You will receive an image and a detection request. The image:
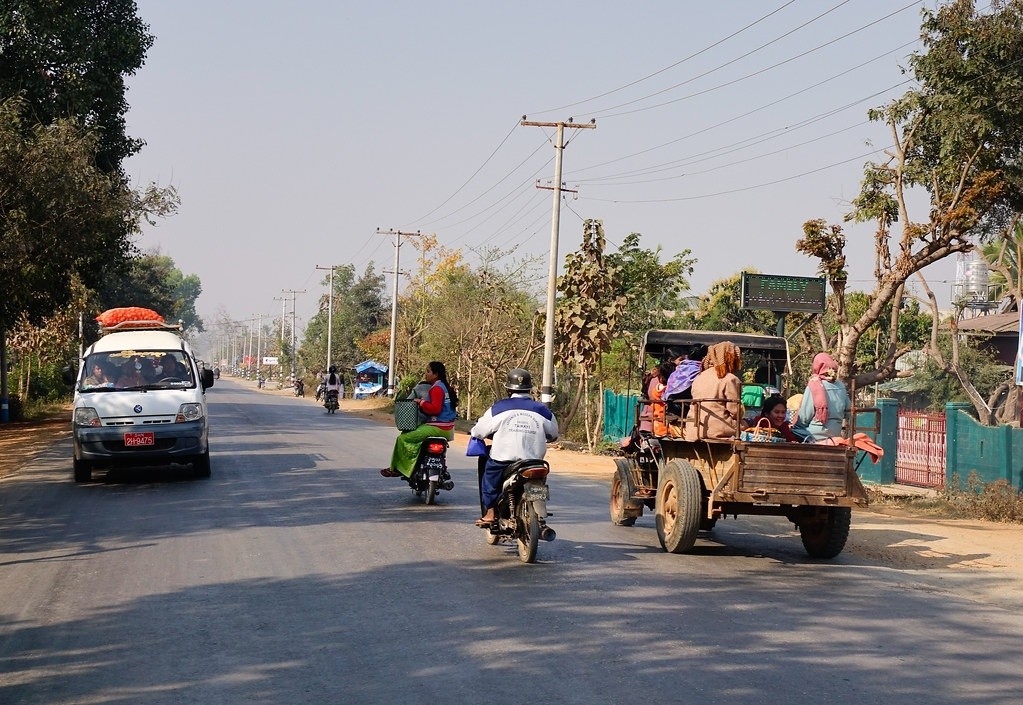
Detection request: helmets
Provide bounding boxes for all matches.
[330,366,337,373]
[505,369,533,391]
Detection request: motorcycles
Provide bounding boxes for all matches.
[258,377,265,389]
[401,399,462,506]
[467,430,556,563]
[292,375,305,397]
[315,380,325,402]
[215,367,221,380]
[319,378,342,413]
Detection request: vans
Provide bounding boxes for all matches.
[72,330,214,482]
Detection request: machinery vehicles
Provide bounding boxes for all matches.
[609,328,882,558]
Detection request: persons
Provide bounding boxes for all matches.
[208,366,370,410]
[83,354,188,389]
[644,340,850,443]
[470,369,559,525]
[406,377,432,425]
[380,362,461,477]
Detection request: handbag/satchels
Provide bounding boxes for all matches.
[739,417,786,443]
[466,437,487,456]
[393,389,420,430]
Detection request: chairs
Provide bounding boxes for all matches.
[161,363,187,381]
[102,362,117,376]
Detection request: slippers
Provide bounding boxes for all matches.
[475,517,494,524]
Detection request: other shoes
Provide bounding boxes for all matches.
[381,468,401,477]
